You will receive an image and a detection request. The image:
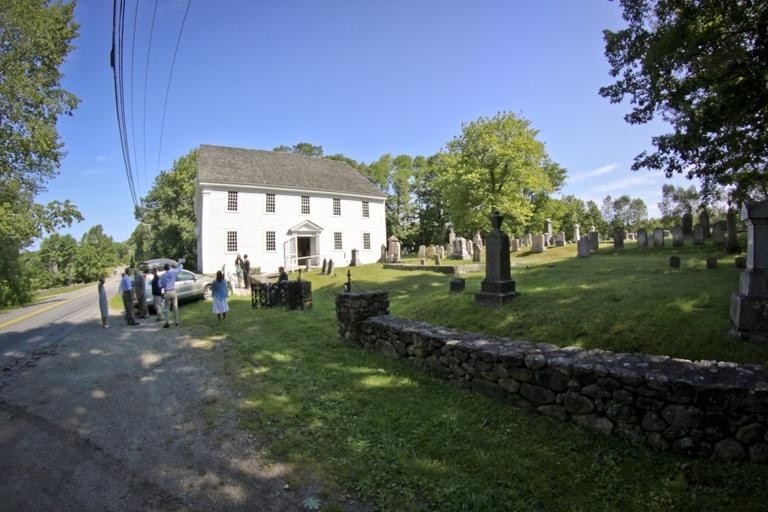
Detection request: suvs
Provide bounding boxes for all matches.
[131,258,216,309]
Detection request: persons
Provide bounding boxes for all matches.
[118,273,128,321]
[235,254,244,288]
[121,268,141,325]
[210,271,230,320]
[134,268,150,320]
[242,255,250,289]
[158,262,184,328]
[151,268,166,321]
[98,275,112,328]
[276,267,288,293]
